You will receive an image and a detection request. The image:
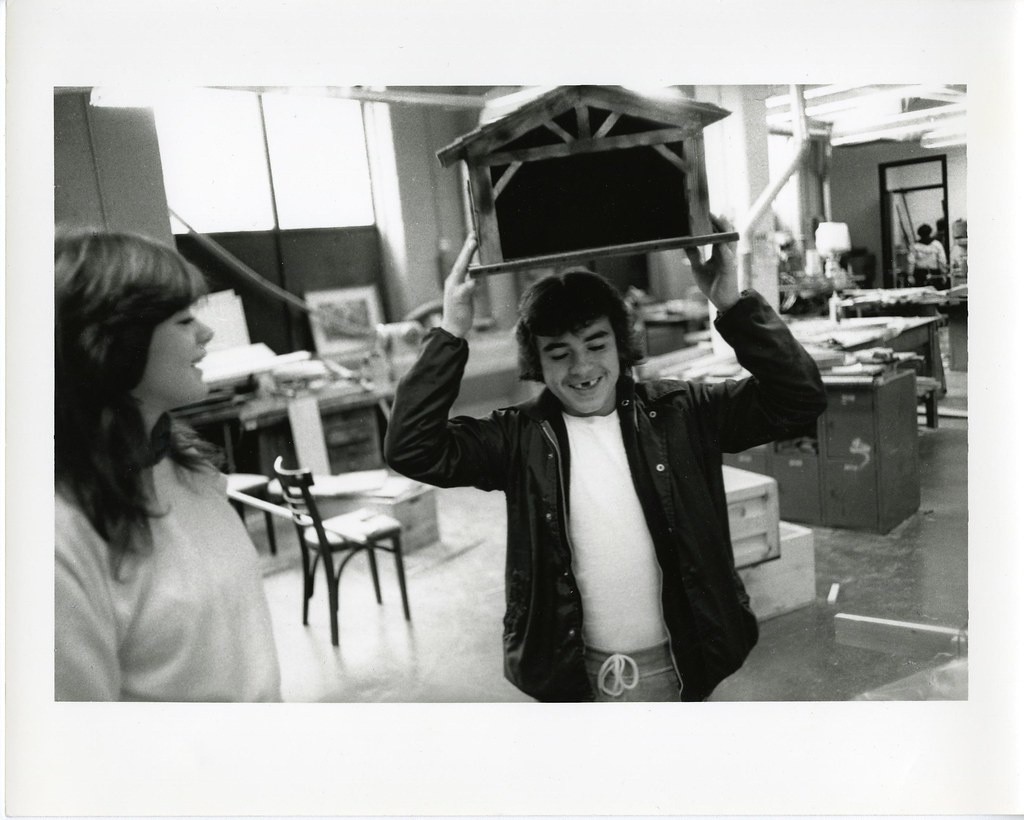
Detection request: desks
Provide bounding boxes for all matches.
[177,383,398,480]
[223,474,278,557]
[643,285,940,428]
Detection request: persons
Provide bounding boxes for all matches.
[54,230,279,703]
[908,225,947,290]
[385,209,826,702]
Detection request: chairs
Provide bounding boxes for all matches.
[274,456,412,646]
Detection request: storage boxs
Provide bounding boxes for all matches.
[363,478,439,555]
[722,464,816,622]
[321,408,382,475]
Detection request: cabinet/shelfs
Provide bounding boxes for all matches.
[722,369,922,534]
